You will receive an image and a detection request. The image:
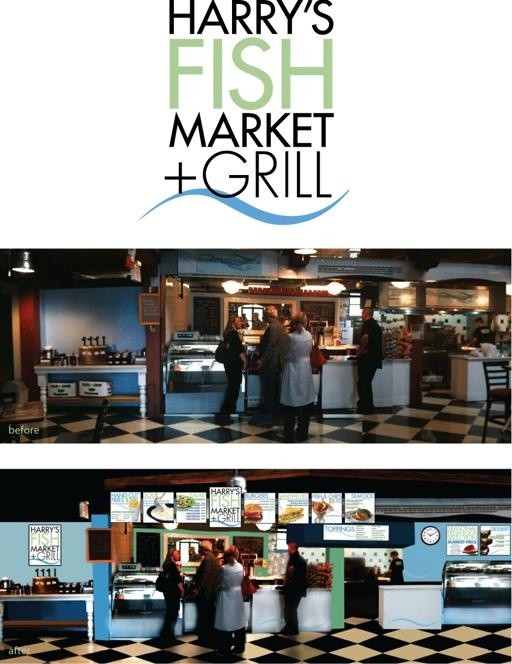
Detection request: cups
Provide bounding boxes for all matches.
[108,351,130,364]
[59,583,78,593]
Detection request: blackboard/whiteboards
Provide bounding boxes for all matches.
[190,293,224,337]
[86,528,112,562]
[139,292,161,325]
[233,535,264,557]
[297,297,339,326]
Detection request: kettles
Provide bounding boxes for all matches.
[40,350,54,359]
[0,577,9,589]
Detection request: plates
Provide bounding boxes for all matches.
[176,495,195,511]
[349,511,372,524]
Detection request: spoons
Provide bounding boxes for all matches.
[154,493,166,504]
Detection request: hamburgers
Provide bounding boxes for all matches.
[244,503,264,524]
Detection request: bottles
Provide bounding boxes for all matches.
[24,583,30,595]
[70,352,77,366]
[15,583,21,594]
[62,353,67,365]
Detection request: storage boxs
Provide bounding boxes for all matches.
[46,381,76,399]
[77,379,113,399]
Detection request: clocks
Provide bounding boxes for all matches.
[420,526,441,545]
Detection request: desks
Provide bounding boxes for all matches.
[377,584,443,632]
[1,593,94,645]
[449,353,511,402]
[33,362,147,420]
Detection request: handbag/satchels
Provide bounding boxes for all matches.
[215,342,230,363]
[310,345,326,368]
[242,576,256,595]
[155,572,166,592]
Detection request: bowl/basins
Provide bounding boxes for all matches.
[147,503,174,522]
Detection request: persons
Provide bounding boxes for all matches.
[176,538,247,654]
[155,548,187,646]
[280,543,308,636]
[470,317,495,347]
[211,305,319,443]
[381,550,405,584]
[355,305,384,415]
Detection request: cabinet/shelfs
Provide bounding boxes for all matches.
[111,565,333,641]
[441,558,512,625]
[165,338,411,424]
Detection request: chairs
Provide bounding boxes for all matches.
[481,356,511,441]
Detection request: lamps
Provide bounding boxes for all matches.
[220,277,247,295]
[391,280,411,290]
[12,249,35,274]
[323,280,347,296]
[300,284,346,291]
[249,284,270,288]
[162,523,178,530]
[294,249,319,254]
[229,470,248,494]
[255,524,273,531]
